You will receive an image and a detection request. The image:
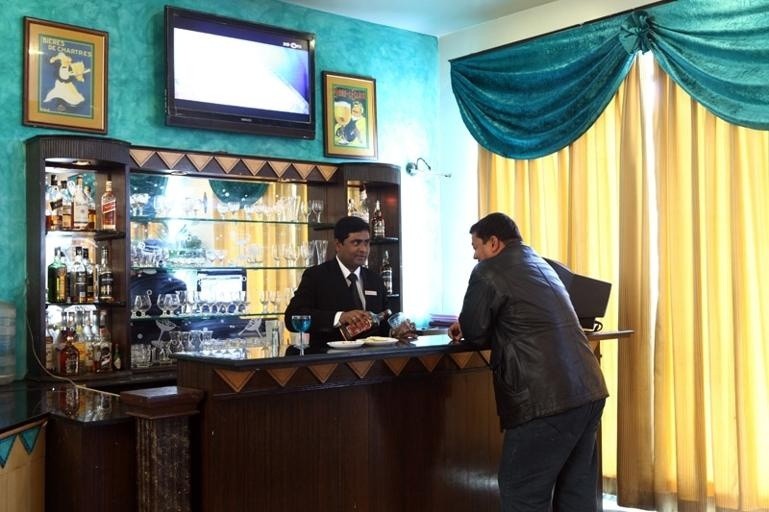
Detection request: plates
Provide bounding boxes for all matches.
[357,338,399,346]
[431,314,459,323]
[327,342,364,348]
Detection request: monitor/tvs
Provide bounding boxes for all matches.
[542,256,612,331]
[162,2,316,143]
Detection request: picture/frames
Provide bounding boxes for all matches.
[21,14,109,136]
[320,70,380,162]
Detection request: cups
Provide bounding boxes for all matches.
[131,344,153,367]
[387,312,412,337]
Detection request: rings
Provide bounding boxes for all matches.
[351,316,357,320]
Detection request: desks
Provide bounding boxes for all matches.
[0,386,47,511]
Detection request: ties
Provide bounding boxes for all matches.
[347,273,363,310]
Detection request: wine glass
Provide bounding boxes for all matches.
[202,338,248,358]
[290,315,312,349]
[130,193,329,319]
[151,319,175,348]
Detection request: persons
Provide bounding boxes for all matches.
[284,216,416,348]
[447,214,610,512]
[129,239,191,343]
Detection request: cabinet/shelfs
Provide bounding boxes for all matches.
[21,135,403,385]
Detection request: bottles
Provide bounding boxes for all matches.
[159,349,165,361]
[347,183,393,295]
[167,331,214,351]
[45,172,122,376]
[47,384,112,421]
[339,309,392,341]
